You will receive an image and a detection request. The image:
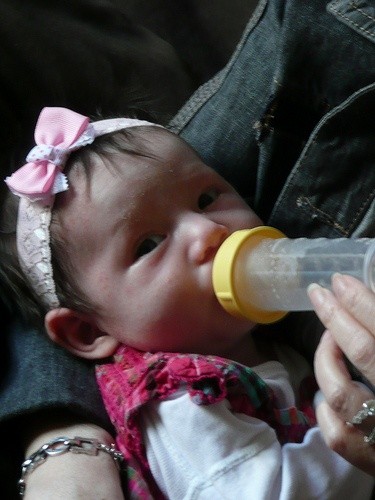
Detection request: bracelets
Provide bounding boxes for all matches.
[18,437,125,495]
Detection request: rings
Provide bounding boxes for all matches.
[345,400,375,444]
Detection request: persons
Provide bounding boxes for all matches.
[0,0,375,500]
[1,112,375,500]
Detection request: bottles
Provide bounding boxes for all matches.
[212,225,374,324]
